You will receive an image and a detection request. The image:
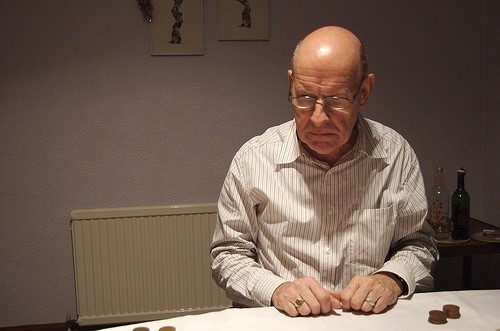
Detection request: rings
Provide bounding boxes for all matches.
[294,297,303,308]
[365,298,375,307]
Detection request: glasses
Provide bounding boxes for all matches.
[288,73,365,110]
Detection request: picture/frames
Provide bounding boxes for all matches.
[149,0,206,56]
[217,0,271,41]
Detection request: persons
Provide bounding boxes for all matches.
[210,25,439,318]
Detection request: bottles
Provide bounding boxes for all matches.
[451,169,470,241]
[429,166,450,241]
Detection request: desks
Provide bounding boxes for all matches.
[428,213,500,289]
[97,281,500,331]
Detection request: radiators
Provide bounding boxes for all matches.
[68,201,242,327]
